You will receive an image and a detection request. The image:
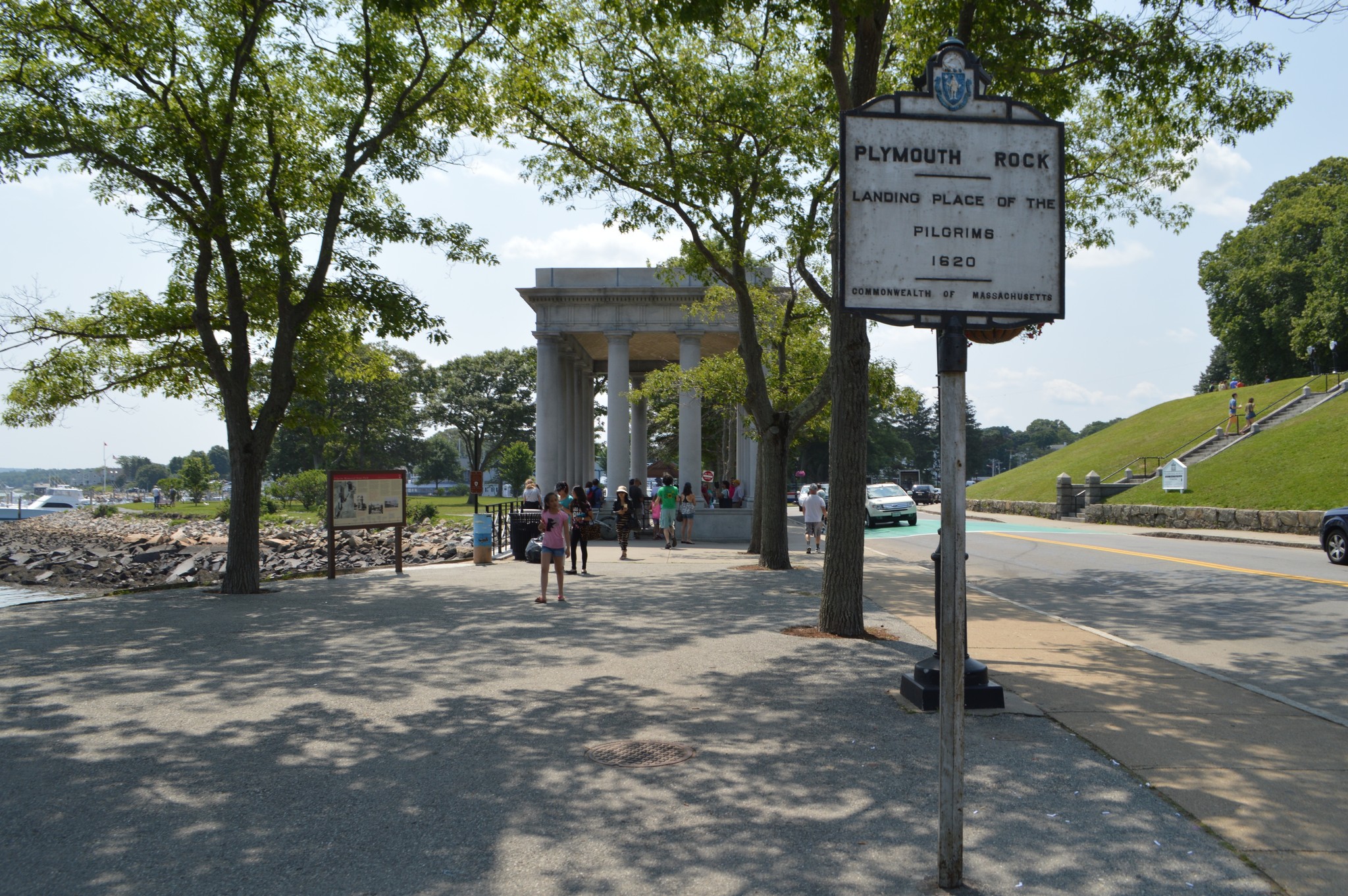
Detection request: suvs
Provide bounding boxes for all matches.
[865,482,917,529]
[910,484,939,505]
[820,483,829,507]
[796,484,811,512]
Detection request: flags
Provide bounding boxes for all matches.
[113,456,118,460]
[104,443,107,446]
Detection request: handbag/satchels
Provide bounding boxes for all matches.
[522,503,526,509]
[719,489,732,508]
[579,515,602,541]
[676,511,683,522]
[641,502,645,515]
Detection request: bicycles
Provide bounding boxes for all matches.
[569,500,619,541]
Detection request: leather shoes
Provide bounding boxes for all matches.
[581,571,587,574]
[565,571,577,574]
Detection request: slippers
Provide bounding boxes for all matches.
[558,596,565,601]
[534,597,547,603]
[620,555,626,559]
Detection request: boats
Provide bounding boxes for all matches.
[0,441,297,519]
[405,436,536,496]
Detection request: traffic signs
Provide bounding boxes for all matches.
[470,471,483,495]
[839,108,1065,321]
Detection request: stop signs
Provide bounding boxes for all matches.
[702,470,714,483]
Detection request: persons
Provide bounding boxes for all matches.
[87,484,176,508]
[653,474,680,549]
[1224,393,1245,436]
[680,482,696,544]
[647,493,663,540]
[801,483,828,554]
[534,492,571,603]
[1264,375,1270,383]
[554,481,574,572]
[565,485,594,574]
[629,477,645,539]
[585,477,744,509]
[523,479,541,510]
[1239,397,1256,435]
[611,486,635,560]
[816,483,826,534]
[535,484,544,510]
[1208,378,1243,393]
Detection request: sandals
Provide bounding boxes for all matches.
[665,543,671,549]
[671,536,677,547]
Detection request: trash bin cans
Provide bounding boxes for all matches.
[472,514,493,563]
[508,513,542,561]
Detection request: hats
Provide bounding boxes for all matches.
[634,478,642,485]
[730,477,735,479]
[1211,377,1236,384]
[554,481,565,492]
[732,479,740,484]
[684,482,692,488]
[809,483,818,491]
[616,486,629,496]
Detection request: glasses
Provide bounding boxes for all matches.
[556,488,562,492]
[618,492,625,494]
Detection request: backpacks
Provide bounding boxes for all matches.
[587,487,599,505]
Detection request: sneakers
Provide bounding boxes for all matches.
[806,546,811,554]
[815,548,821,553]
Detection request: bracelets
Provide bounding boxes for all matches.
[538,529,543,531]
[561,506,564,509]
[582,517,584,520]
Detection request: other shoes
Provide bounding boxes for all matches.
[1240,431,1245,435]
[681,540,686,543]
[686,541,695,544]
[638,520,641,526]
[1224,433,1231,436]
[633,533,664,540]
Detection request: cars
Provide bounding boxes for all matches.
[1319,508,1348,565]
[934,488,941,503]
[965,481,976,488]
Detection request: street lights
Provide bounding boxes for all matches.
[986,458,999,477]
[975,469,979,483]
[934,465,941,488]
[793,453,799,505]
[996,466,1006,474]
